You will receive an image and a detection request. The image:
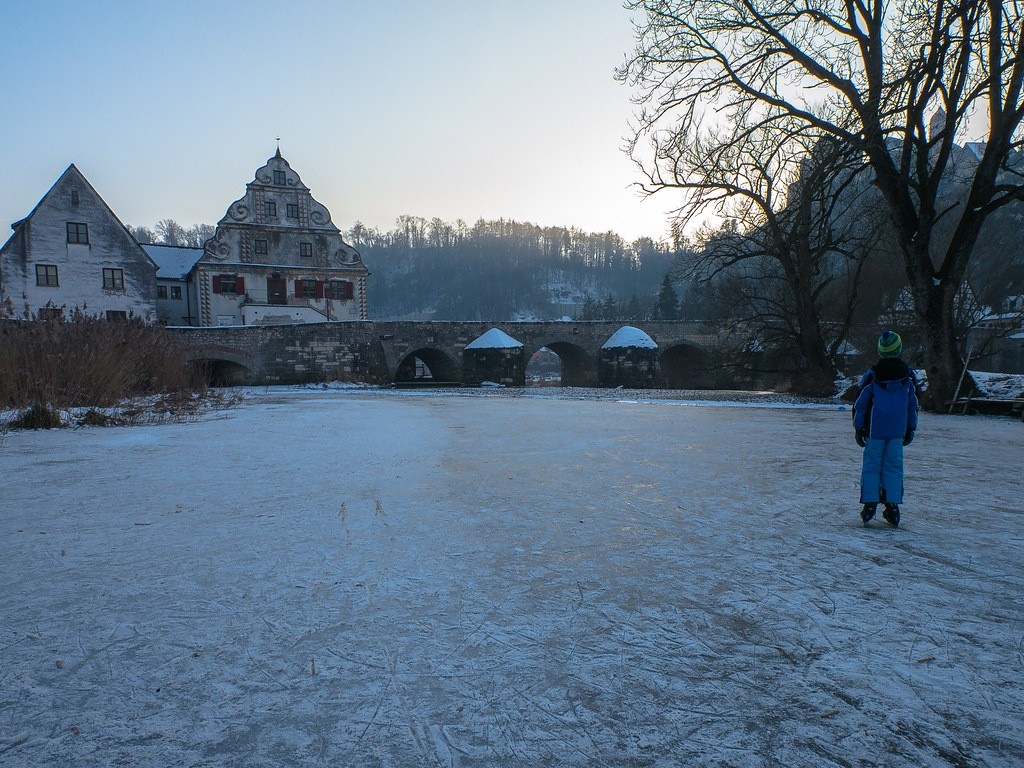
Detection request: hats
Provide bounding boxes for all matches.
[877,330,902,358]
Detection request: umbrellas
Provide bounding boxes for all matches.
[463,328,524,350]
[601,325,659,350]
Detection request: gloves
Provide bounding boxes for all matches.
[855,429,868,447]
[903,431,914,446]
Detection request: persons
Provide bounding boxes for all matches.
[852,331,921,528]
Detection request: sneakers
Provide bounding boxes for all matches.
[881,504,900,528]
[860,503,877,526]
